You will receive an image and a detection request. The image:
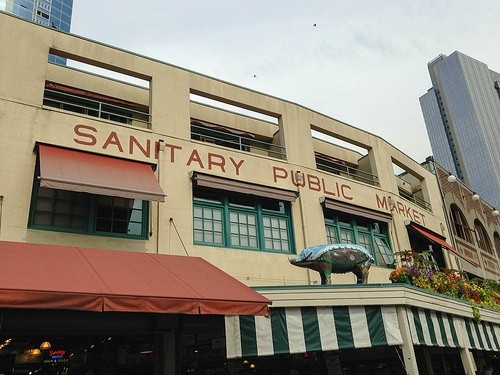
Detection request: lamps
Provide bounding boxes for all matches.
[438,172,455,184]
[388,195,394,217]
[484,207,499,217]
[295,171,301,197]
[31,349,41,356]
[468,191,479,201]
[40,342,51,350]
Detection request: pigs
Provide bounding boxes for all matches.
[289,244,374,286]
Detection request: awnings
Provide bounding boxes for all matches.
[0,241,276,318]
[406,220,478,269]
[37,144,165,202]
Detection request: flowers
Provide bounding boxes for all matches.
[388,248,499,308]
